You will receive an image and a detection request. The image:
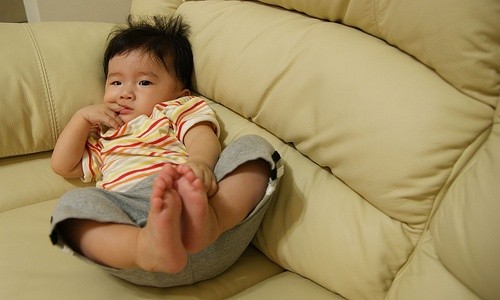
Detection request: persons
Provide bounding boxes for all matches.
[48,14,285,288]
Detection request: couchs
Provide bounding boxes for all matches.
[0,1,500,300]
